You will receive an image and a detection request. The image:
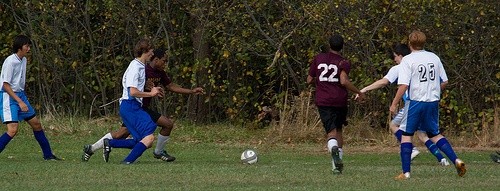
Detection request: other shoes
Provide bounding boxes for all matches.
[410,146,420,161]
[44,154,65,162]
[489,150,500,163]
[455,158,466,177]
[331,146,344,170]
[394,173,411,181]
[332,167,341,175]
[441,158,450,167]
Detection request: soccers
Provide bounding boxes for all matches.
[241,150,257,165]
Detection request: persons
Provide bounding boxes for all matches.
[81,44,203,162]
[103,39,164,165]
[306,31,367,176]
[0,35,64,161]
[354,42,451,166]
[388,31,467,180]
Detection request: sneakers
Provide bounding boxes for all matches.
[82,144,94,163]
[103,138,112,162]
[153,149,176,162]
